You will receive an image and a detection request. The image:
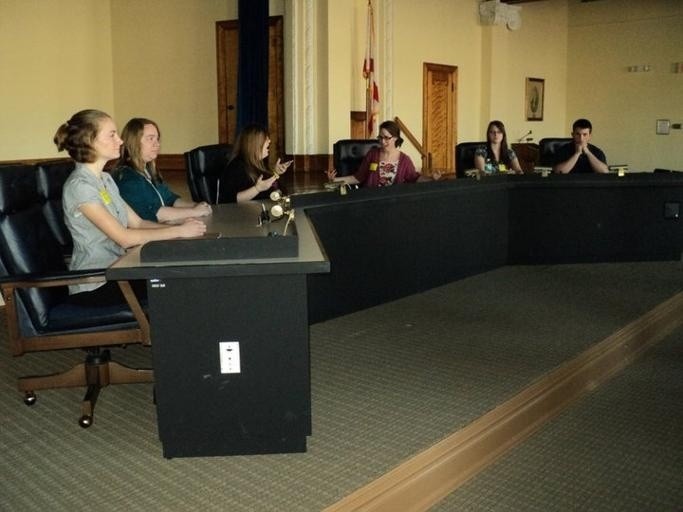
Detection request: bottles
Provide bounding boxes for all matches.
[484,158,493,175]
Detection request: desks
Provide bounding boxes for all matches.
[107,169,682,458]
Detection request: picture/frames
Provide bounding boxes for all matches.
[526,77,545,121]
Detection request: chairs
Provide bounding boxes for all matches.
[36,157,127,249]
[1,164,157,428]
[334,140,380,177]
[184,143,240,204]
[455,142,487,179]
[539,139,580,175]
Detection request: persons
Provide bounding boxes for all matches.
[323,121,446,185]
[52,108,207,306]
[474,120,525,176]
[108,117,213,223]
[216,125,294,203]
[553,118,609,175]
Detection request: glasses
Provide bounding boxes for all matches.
[377,135,394,140]
